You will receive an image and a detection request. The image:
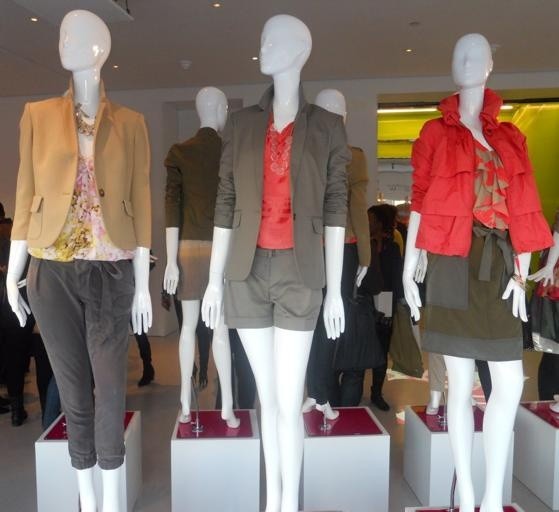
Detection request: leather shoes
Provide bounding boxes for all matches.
[370,387,391,411]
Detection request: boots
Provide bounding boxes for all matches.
[36,360,62,426]
[137,342,155,387]
[8,364,27,427]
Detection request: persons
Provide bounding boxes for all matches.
[5,6,154,512]
[527,208,559,399]
[200,12,347,512]
[402,30,532,512]
[161,82,244,429]
[303,86,372,421]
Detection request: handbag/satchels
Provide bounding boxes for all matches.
[336,295,385,371]
[388,300,424,378]
